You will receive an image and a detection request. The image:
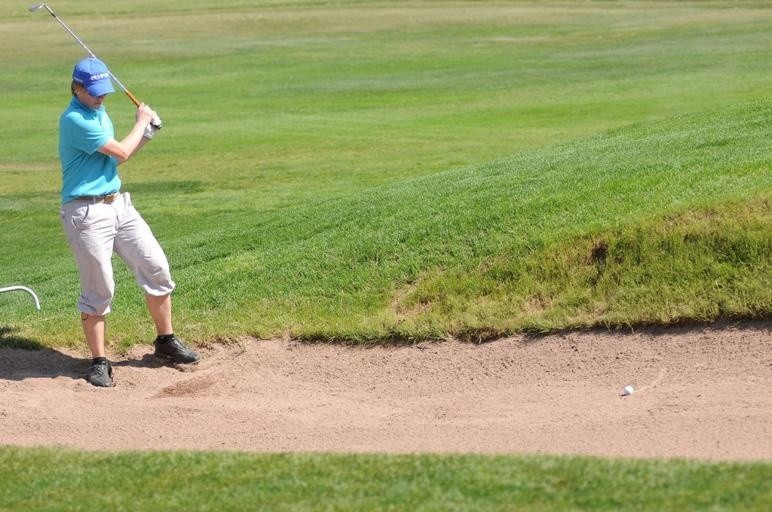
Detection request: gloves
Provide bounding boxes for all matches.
[143,105,161,140]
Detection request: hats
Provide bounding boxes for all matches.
[72,57,115,97]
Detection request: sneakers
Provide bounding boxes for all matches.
[152,336,201,364]
[87,357,113,388]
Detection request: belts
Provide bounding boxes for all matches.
[75,192,120,204]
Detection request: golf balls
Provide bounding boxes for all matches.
[624,386,633,395]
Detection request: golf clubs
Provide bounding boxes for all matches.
[29,3,161,132]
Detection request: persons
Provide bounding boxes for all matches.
[57,55,200,391]
